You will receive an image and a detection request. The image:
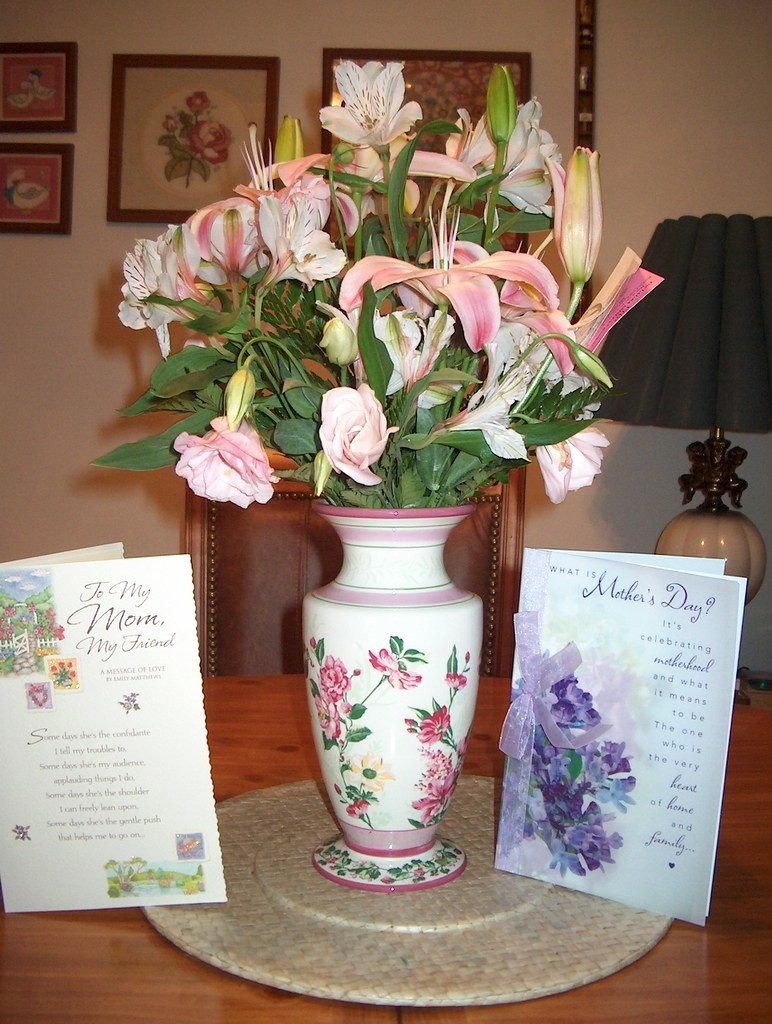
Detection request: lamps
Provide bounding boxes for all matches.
[593,213,771,707]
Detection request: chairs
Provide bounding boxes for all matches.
[181,446,527,677]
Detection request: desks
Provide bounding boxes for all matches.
[0,675,772,1023]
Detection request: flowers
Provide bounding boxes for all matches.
[89,57,615,509]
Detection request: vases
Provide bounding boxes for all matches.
[300,498,485,891]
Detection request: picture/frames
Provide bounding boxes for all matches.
[106,53,282,224]
[0,40,77,134]
[320,46,532,255]
[0,142,75,236]
